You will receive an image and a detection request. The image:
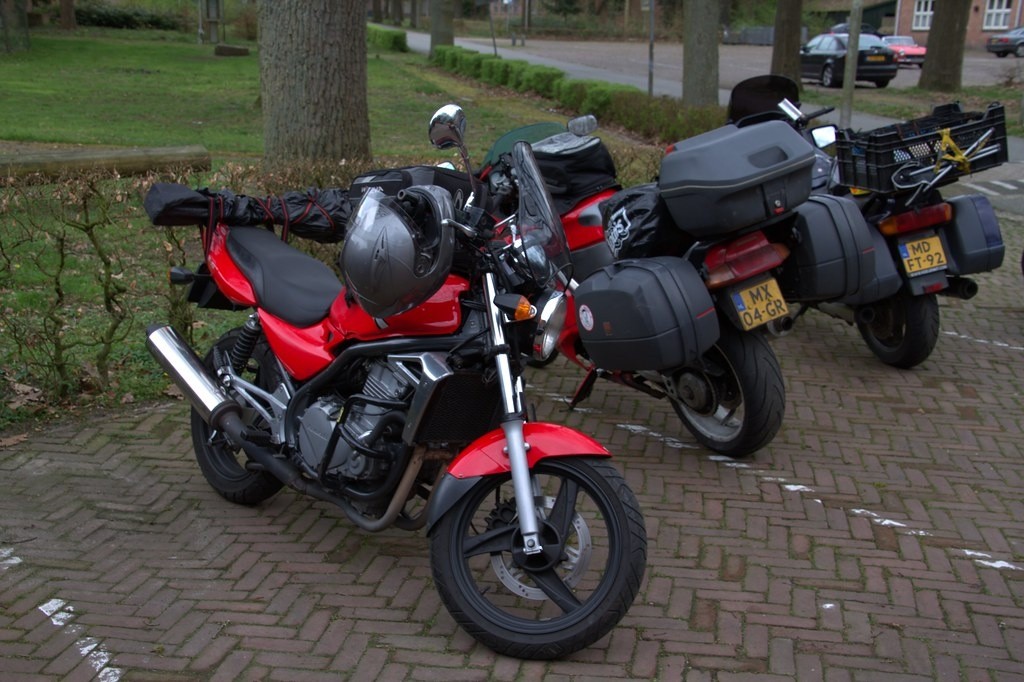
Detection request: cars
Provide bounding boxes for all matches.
[985,26,1024,58]
[802,33,898,89]
[829,21,883,37]
[881,35,927,68]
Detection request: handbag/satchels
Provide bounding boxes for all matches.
[598,183,677,263]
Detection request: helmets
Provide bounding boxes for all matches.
[339,185,455,319]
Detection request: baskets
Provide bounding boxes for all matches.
[835,103,1009,194]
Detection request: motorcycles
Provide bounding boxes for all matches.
[726,76,1010,370]
[142,105,649,663]
[478,114,817,458]
